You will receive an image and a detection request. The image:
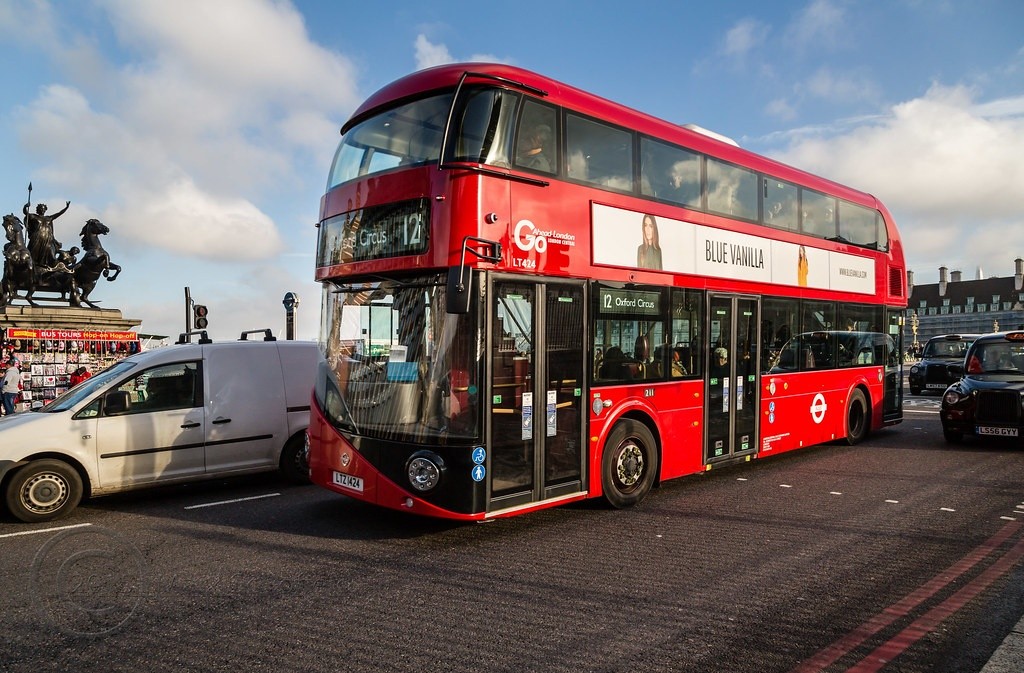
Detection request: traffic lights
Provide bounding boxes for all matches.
[194,304,208,330]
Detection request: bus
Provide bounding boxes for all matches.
[306,59,909,521]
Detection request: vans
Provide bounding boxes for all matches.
[0,329,329,526]
[768,331,898,369]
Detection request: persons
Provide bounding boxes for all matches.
[23,199,71,267]
[597,348,634,378]
[0,371,8,416]
[672,350,688,377]
[984,349,1016,369]
[131,371,183,411]
[798,245,808,287]
[904,342,924,362]
[594,349,602,374]
[637,213,663,271]
[709,347,730,409]
[823,318,860,367]
[517,131,834,236]
[2,359,19,415]
[56,246,82,270]
[70,367,91,389]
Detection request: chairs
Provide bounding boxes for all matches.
[599,340,876,379]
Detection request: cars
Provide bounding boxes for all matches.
[939,330,1024,444]
[909,334,1013,394]
